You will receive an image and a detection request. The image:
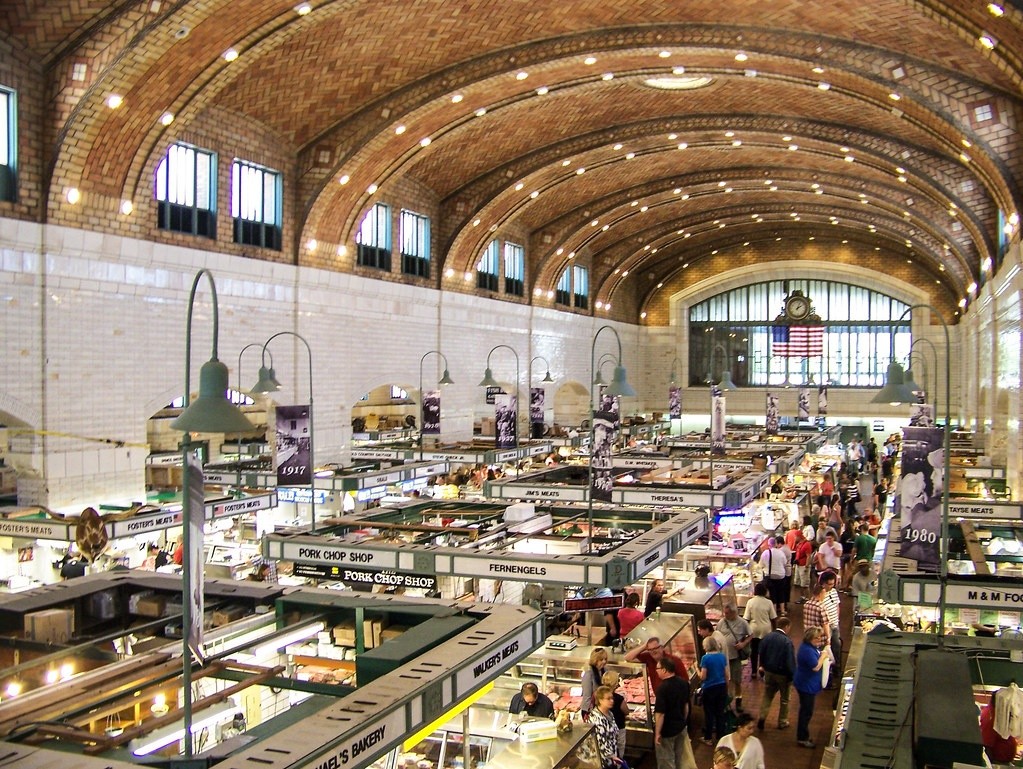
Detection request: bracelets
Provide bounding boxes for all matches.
[830,545,835,549]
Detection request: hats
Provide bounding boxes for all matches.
[857,559,869,566]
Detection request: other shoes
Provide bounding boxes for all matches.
[736,697,744,713]
[781,611,787,617]
[780,722,790,729]
[752,674,758,680]
[798,739,815,748]
[699,736,712,746]
[795,597,808,605]
[757,717,765,730]
[759,668,764,677]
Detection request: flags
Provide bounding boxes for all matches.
[772,324,823,357]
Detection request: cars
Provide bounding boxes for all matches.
[873,419,884,431]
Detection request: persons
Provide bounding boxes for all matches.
[689,618,729,746]
[836,435,879,475]
[224,517,240,538]
[741,582,778,680]
[59,552,89,582]
[757,534,793,616]
[803,585,841,690]
[820,572,844,676]
[814,461,888,515]
[789,627,827,748]
[580,647,630,769]
[546,447,562,466]
[879,430,903,479]
[714,603,754,712]
[508,683,554,719]
[411,490,419,499]
[599,577,669,646]
[708,711,767,769]
[243,563,270,583]
[425,461,510,491]
[783,502,843,603]
[493,578,525,606]
[623,635,698,768]
[981,691,1017,764]
[174,533,184,565]
[147,545,172,572]
[837,506,882,608]
[757,617,797,730]
[967,623,999,637]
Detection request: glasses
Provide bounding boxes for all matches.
[648,644,662,652]
[655,667,665,671]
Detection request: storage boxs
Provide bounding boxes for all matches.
[332,616,409,649]
[129,590,247,628]
[23,608,74,644]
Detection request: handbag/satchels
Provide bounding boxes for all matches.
[738,645,750,660]
[762,576,773,589]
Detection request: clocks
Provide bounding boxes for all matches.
[773,289,823,326]
